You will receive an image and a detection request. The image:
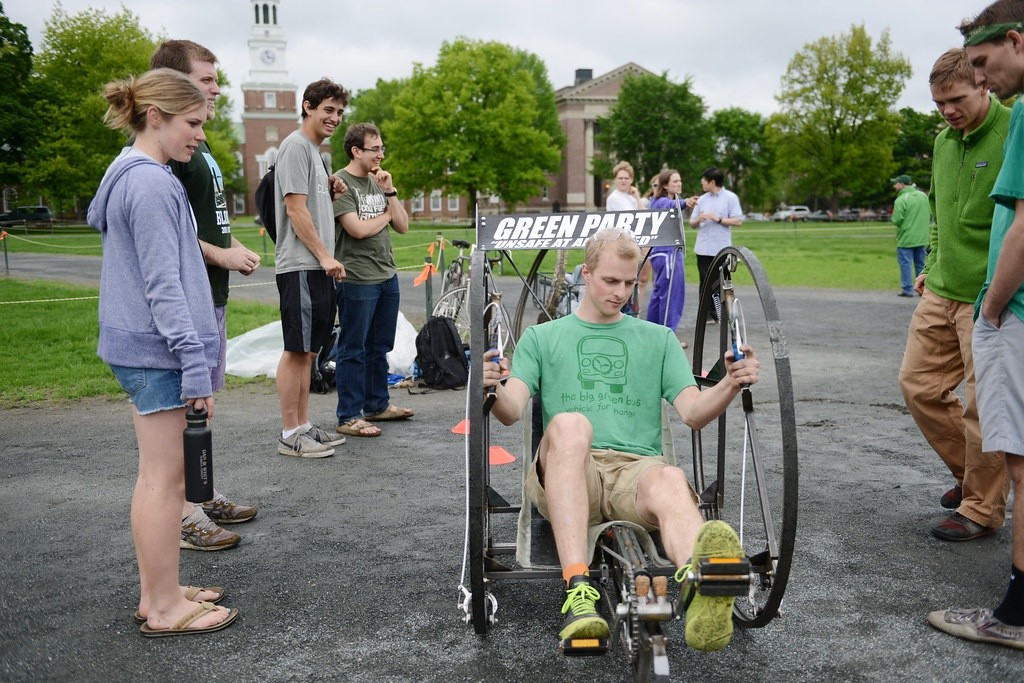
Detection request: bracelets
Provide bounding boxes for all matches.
[717,218,722,224]
[384,187,397,197]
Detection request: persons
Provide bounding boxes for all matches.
[332,123,415,437]
[274,80,348,457]
[638,175,659,310]
[929,0,1024,647]
[891,175,932,297]
[646,170,699,348]
[483,228,760,652]
[151,40,261,551]
[606,161,643,210]
[898,47,1012,540]
[86,68,239,637]
[689,167,743,325]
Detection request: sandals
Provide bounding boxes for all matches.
[334,418,382,436]
[364,404,414,421]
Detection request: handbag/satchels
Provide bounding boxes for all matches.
[310,324,342,395]
[255,152,336,244]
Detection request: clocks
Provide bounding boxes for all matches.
[261,49,276,65]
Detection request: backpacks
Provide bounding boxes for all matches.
[414,316,469,391]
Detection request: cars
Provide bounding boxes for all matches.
[746,205,893,223]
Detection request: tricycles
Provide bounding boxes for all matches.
[458,196,798,683]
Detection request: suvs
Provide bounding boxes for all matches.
[0,206,54,228]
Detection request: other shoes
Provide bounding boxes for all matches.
[678,340,687,348]
[898,292,914,297]
[706,312,720,325]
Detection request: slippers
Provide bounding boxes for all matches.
[139,601,239,637]
[134,586,225,623]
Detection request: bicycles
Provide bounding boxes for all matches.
[432,240,512,354]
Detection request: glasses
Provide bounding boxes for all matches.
[360,145,387,155]
[652,184,659,188]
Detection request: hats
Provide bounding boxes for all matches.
[890,175,911,185]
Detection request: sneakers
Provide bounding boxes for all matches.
[179,506,241,550]
[939,484,963,509]
[930,513,995,540]
[927,606,1024,649]
[202,495,256,523]
[675,521,744,650]
[556,574,610,653]
[277,428,336,458]
[308,425,347,446]
